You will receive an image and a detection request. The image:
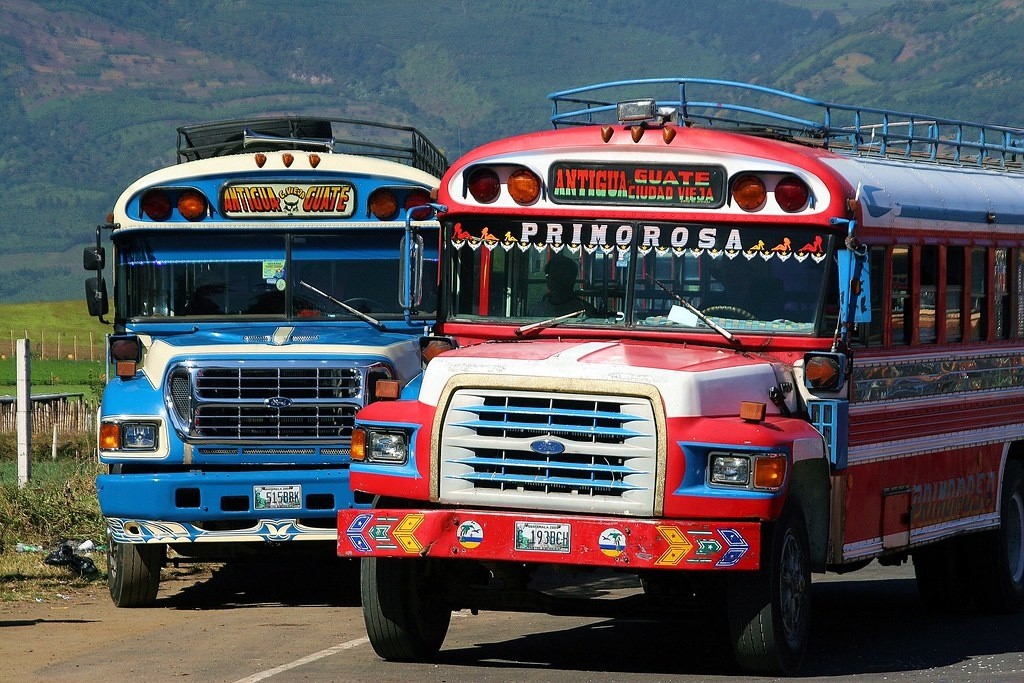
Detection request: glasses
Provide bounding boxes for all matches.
[545,272,571,282]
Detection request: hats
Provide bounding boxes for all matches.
[543,255,578,279]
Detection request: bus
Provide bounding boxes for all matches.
[83,115,477,610]
[334,72,1024,678]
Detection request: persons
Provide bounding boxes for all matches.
[527,254,600,318]
